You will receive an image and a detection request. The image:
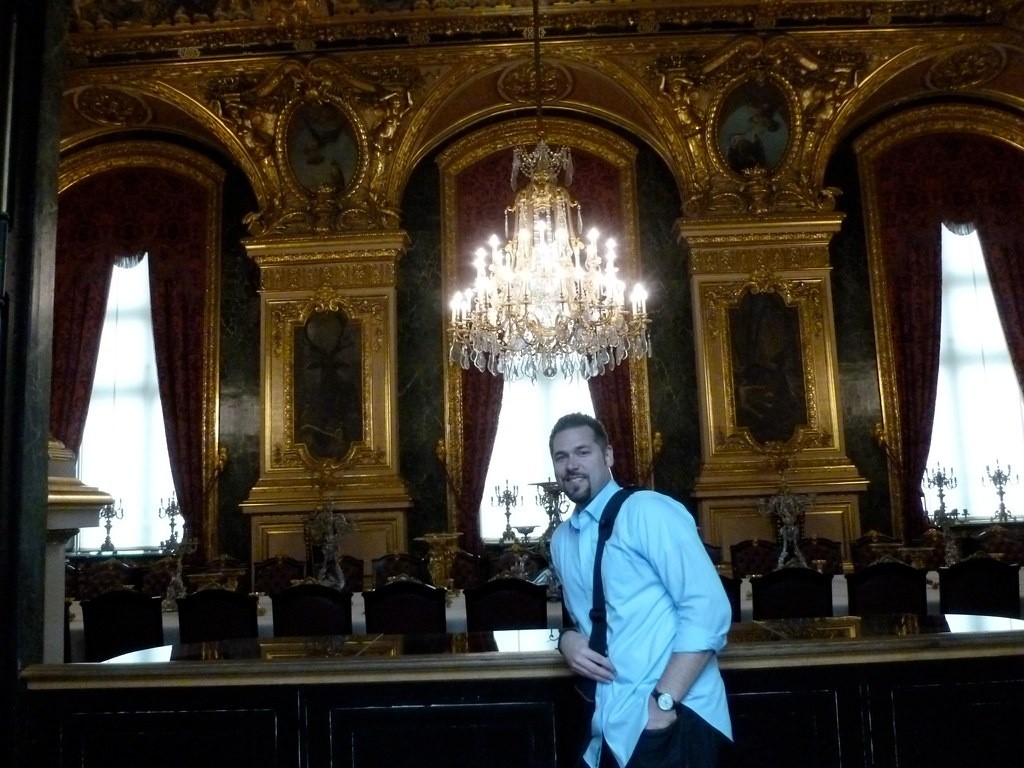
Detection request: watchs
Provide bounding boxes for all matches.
[650,687,679,711]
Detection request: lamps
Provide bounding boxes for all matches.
[447,0,654,386]
[98,497,124,554]
[981,457,1019,522]
[922,461,958,526]
[158,489,181,552]
[490,478,524,545]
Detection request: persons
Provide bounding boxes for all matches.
[547,413,734,768]
[369,92,414,190]
[654,73,708,169]
[800,68,862,172]
[208,100,281,200]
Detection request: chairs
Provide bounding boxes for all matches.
[78,529,1024,661]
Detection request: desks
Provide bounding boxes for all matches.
[100,612,1024,663]
[67,566,1024,663]
